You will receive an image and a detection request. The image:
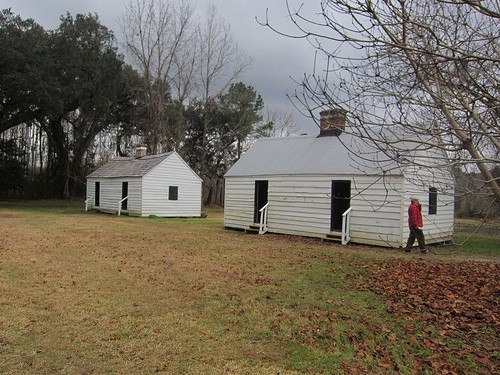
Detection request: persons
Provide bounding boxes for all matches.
[405,196,429,254]
